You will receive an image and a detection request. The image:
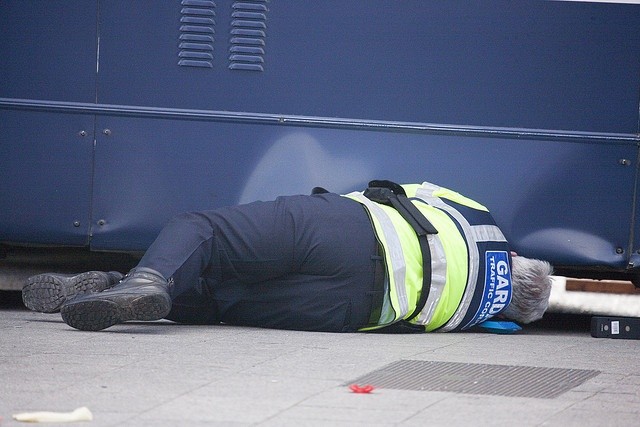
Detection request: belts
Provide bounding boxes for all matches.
[370,247,387,326]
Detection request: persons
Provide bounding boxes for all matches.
[21,180,553,333]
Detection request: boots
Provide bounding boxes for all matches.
[60,267,172,330]
[21,271,123,313]
